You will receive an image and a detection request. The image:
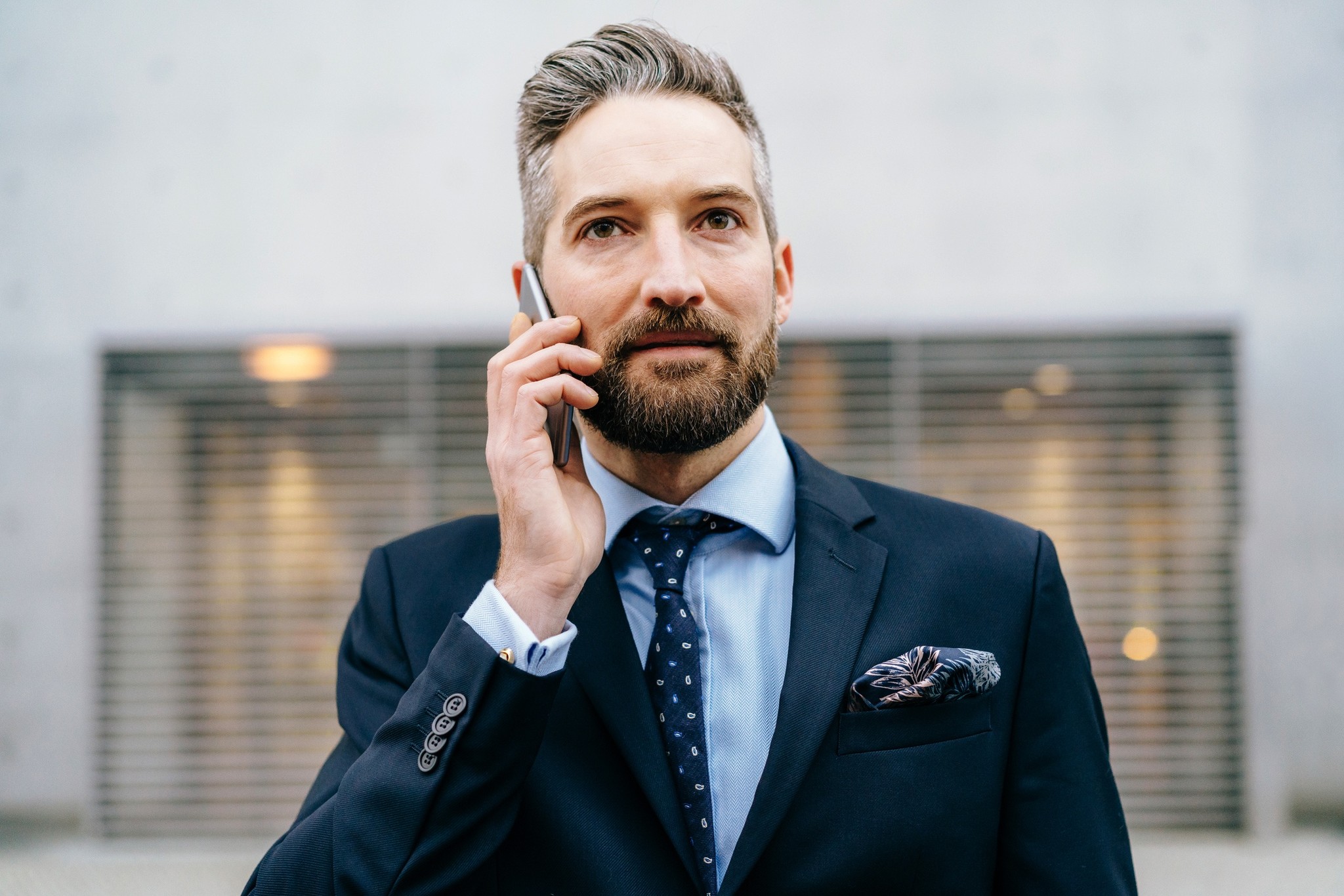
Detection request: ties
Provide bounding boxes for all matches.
[630,514,744,896]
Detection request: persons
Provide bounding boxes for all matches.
[238,23,1138,896]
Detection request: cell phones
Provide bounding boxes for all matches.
[517,264,574,468]
